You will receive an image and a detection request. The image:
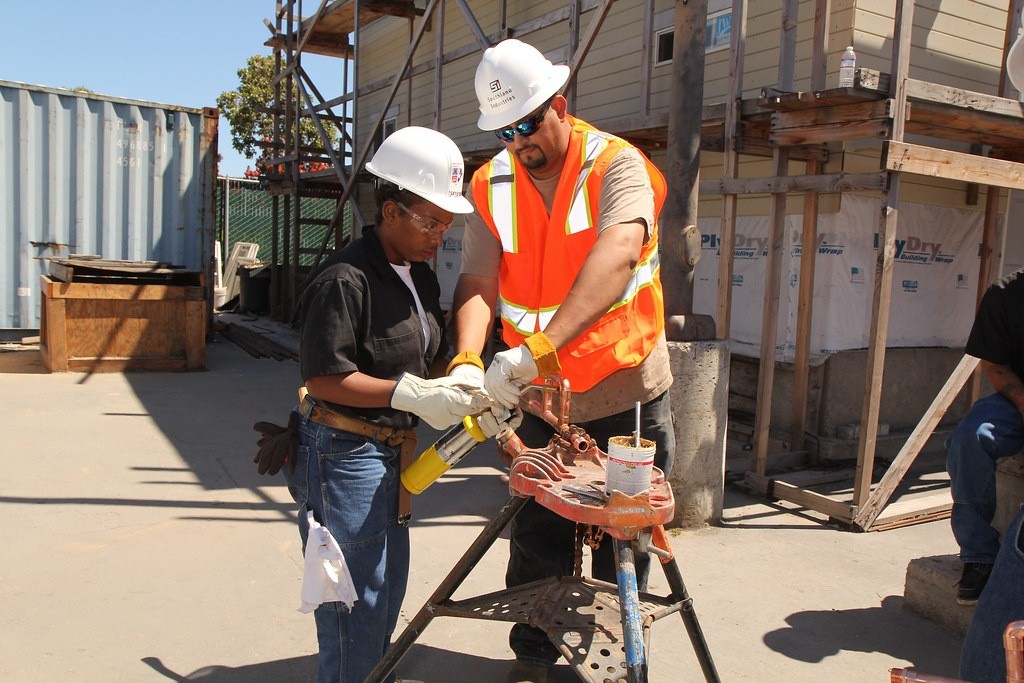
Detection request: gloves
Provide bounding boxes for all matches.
[389,371,494,431]
[484,332,562,409]
[477,406,523,437]
[251,410,299,476]
[445,350,490,397]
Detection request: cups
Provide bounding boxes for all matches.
[603,435,657,499]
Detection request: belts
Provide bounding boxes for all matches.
[299,397,418,524]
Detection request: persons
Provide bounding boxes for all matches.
[446,40,675,662]
[279,126,523,683]
[945,268,1023,683]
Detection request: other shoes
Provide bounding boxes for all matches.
[507,660,550,683]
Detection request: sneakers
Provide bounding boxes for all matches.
[953,563,995,606]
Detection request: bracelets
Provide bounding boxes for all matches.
[447,351,484,376]
[524,332,562,377]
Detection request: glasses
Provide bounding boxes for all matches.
[395,200,453,237]
[496,100,550,143]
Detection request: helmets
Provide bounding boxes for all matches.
[475,39,570,131]
[366,126,475,214]
